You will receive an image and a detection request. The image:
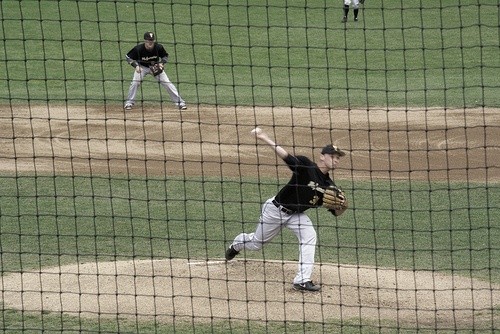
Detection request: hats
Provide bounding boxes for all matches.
[320,144,346,157]
[144,32,155,40]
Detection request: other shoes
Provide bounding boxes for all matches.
[126,105,132,110]
[179,105,187,110]
[354,17,358,21]
[341,16,347,23]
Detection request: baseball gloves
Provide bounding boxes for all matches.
[149,61,164,77]
[322,185,348,216]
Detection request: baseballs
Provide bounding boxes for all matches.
[254,128,261,135]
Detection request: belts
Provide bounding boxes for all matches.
[272,200,295,215]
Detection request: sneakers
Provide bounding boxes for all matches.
[225,244,240,260]
[292,280,321,292]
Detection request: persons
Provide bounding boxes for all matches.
[225,127,349,291]
[124,31,187,110]
[340,0,365,22]
